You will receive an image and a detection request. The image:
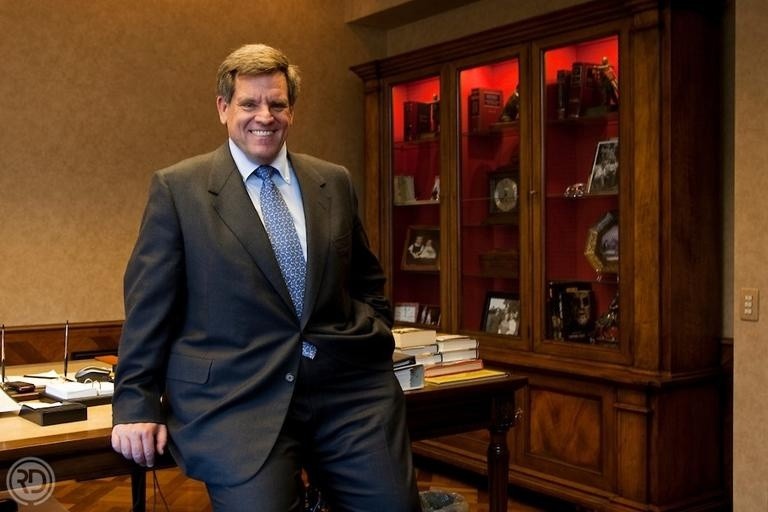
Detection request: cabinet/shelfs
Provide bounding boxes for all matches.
[350,0,727,512]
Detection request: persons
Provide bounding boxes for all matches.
[408,236,436,259]
[487,308,519,336]
[110,43,420,512]
[594,57,619,103]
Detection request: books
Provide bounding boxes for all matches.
[393,326,510,395]
[470,88,503,132]
[547,61,603,117]
[404,100,441,140]
[5,370,116,405]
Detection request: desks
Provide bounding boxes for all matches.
[0,354,529,512]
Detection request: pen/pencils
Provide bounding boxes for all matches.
[23,375,59,379]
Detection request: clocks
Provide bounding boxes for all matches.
[487,165,520,221]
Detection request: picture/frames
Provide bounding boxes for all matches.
[399,223,443,272]
[479,290,522,339]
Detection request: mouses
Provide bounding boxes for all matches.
[75,366,111,383]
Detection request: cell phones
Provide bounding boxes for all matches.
[6,381,35,393]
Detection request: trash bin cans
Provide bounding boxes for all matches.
[418,490,470,511]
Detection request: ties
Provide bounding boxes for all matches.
[254,166,317,361]
